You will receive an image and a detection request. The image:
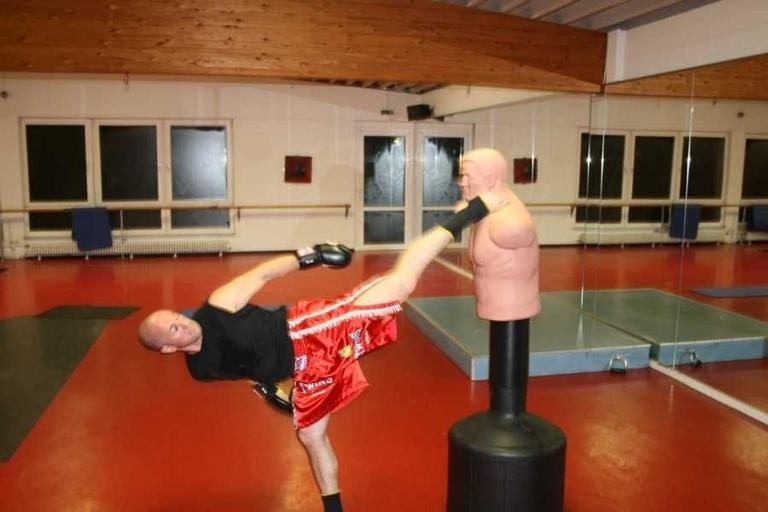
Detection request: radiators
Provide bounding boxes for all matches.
[28,238,229,262]
[578,230,725,250]
[741,230,768,246]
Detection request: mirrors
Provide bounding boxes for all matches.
[419,1,768,427]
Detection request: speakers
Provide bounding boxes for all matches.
[284,155,313,184]
[405,102,430,121]
[515,158,536,185]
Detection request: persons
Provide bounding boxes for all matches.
[138,192,512,512]
[454,149,542,321]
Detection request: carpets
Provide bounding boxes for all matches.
[33,306,140,320]
[0,316,107,463]
[689,286,768,297]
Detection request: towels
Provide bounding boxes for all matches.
[70,209,112,251]
[670,204,703,239]
[747,205,768,230]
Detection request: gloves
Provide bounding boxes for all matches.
[294,243,352,269]
[250,384,294,414]
